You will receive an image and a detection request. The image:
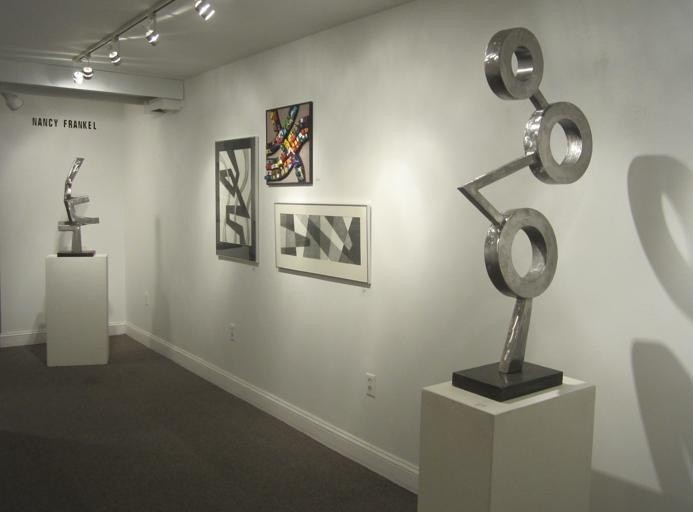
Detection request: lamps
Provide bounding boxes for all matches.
[72,0,216,86]
[0,91,24,111]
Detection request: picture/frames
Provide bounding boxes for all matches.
[216,101,369,286]
